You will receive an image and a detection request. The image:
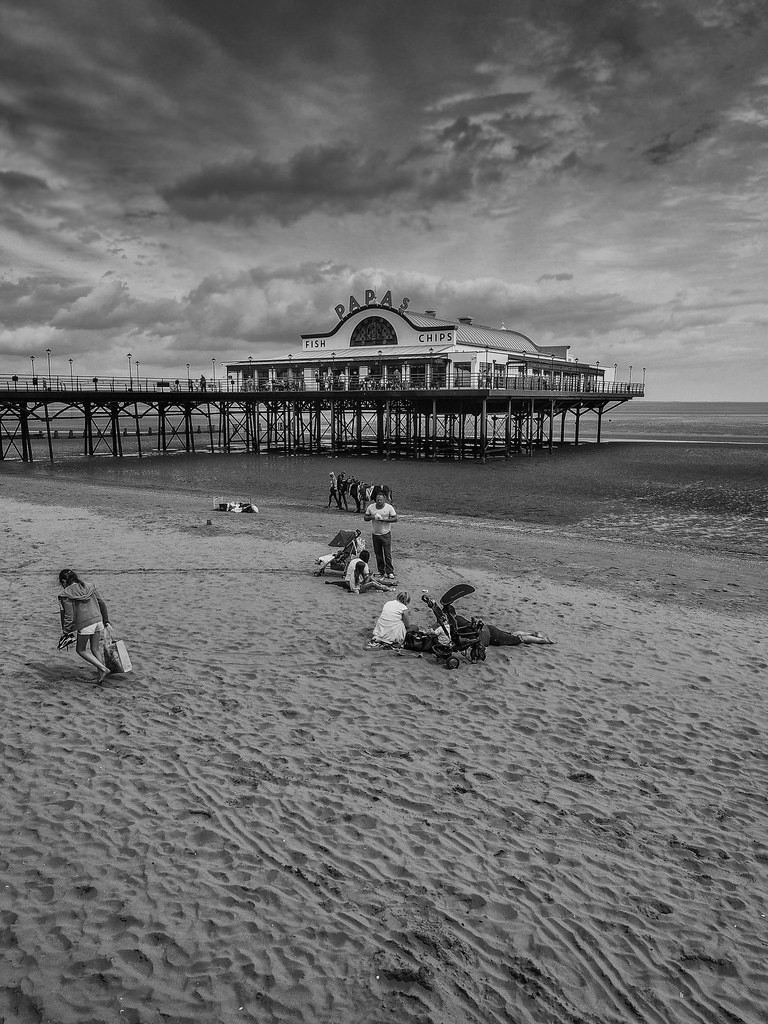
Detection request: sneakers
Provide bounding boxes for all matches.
[69,632,76,642]
[58,635,72,648]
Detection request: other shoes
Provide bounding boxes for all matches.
[390,574,394,578]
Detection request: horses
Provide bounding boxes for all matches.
[341,476,393,513]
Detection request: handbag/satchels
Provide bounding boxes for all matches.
[356,536,366,552]
[104,623,132,674]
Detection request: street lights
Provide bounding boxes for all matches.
[248,356,253,392]
[211,358,216,392]
[597,361,599,391]
[614,364,617,380]
[136,361,140,391]
[126,353,133,391]
[46,349,52,391]
[288,354,292,390]
[378,350,383,389]
[331,352,336,389]
[551,354,555,390]
[186,363,190,391]
[430,348,433,386]
[69,358,74,390]
[575,357,578,391]
[643,368,646,384]
[522,350,527,389]
[30,355,36,391]
[630,365,632,383]
[485,345,490,387]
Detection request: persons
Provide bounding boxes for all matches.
[443,605,557,645]
[344,549,398,593]
[58,569,111,685]
[417,613,452,651]
[323,471,341,508]
[350,560,396,594]
[364,493,398,579]
[373,591,419,645]
[337,470,347,510]
[170,367,597,393]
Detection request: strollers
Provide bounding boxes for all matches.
[314,528,361,580]
[421,594,488,670]
